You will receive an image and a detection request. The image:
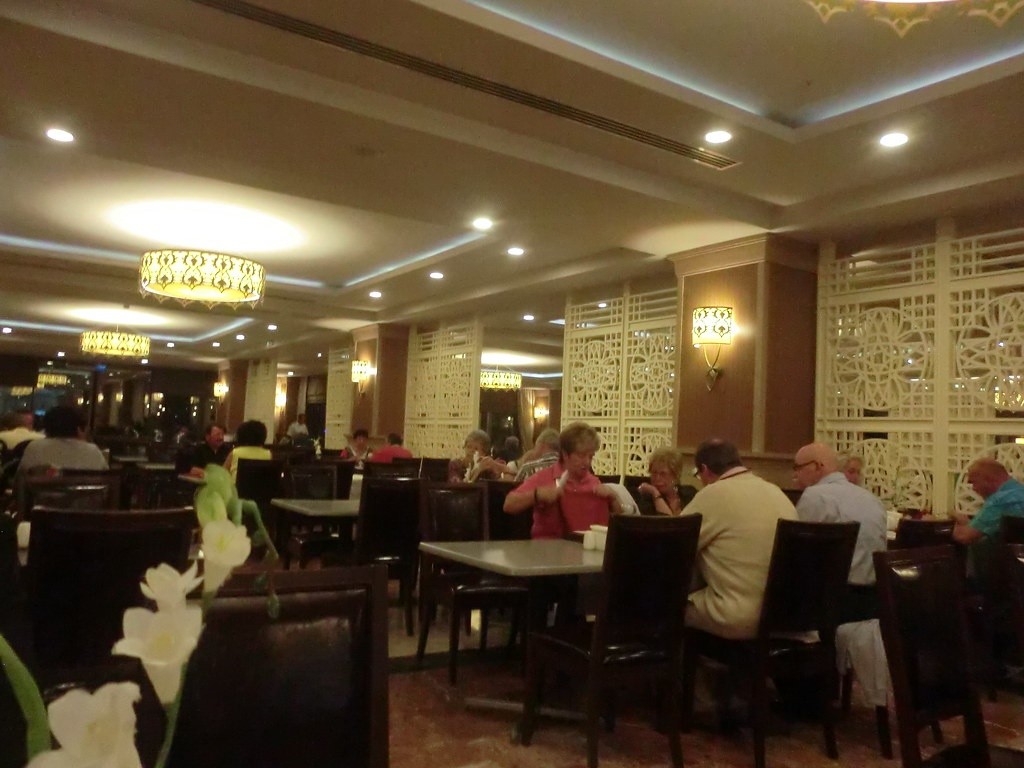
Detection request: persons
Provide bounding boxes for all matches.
[502,424,625,628]
[678,440,819,728]
[793,445,886,612]
[340,429,413,463]
[12,403,111,511]
[449,428,560,482]
[953,458,1024,577]
[637,450,697,515]
[287,413,308,437]
[188,421,271,483]
[839,455,861,484]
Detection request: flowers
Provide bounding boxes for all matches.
[0,462,284,768]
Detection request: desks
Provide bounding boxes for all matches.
[422,539,607,699]
[269,498,359,570]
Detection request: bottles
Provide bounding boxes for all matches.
[583,532,594,550]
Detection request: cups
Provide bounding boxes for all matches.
[886,511,902,530]
[590,525,608,551]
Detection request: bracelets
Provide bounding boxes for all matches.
[652,494,663,499]
[534,487,538,503]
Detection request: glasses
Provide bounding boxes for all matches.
[694,469,702,481]
[792,460,824,471]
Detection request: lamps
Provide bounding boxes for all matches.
[213,382,228,402]
[351,360,373,396]
[137,250,266,309]
[480,362,522,391]
[11,364,71,396]
[80,324,150,356]
[692,306,733,389]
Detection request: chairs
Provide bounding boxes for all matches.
[1,432,1024,768]
